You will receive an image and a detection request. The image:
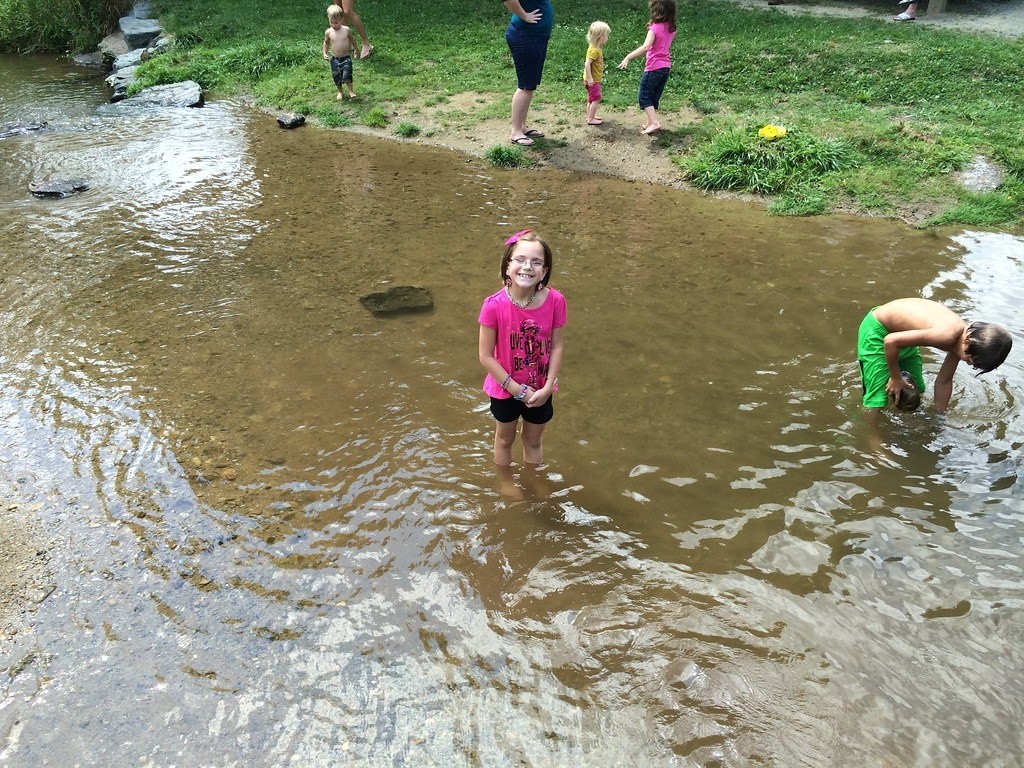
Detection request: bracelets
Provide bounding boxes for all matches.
[501,373,512,389]
[514,383,527,400]
[546,375,560,392]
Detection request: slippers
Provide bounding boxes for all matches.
[524,127,545,137]
[758,123,786,140]
[511,136,535,146]
[894,13,915,22]
[360,43,373,58]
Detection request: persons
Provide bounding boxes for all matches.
[500,0,554,146]
[583,20,612,125]
[617,0,678,136]
[889,370,922,412]
[476,228,568,500]
[855,296,1013,463]
[893,0,919,20]
[322,5,359,101]
[333,0,374,60]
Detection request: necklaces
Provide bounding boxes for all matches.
[504,284,537,309]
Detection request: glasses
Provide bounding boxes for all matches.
[507,257,546,269]
